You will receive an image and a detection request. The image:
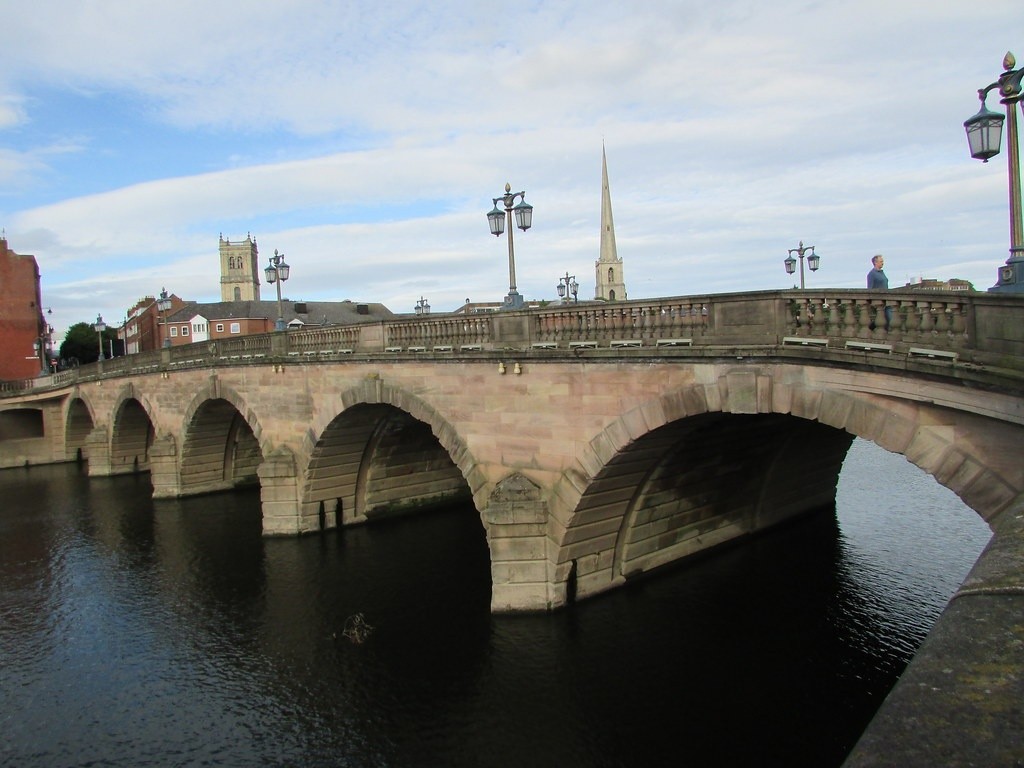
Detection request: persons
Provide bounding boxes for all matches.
[866,254,891,334]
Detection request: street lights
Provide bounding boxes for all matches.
[157,286,173,349]
[413,295,431,317]
[263,248,290,331]
[486,182,534,311]
[32,337,48,378]
[94,313,107,363]
[962,50,1024,295]
[557,272,579,304]
[783,240,820,289]
